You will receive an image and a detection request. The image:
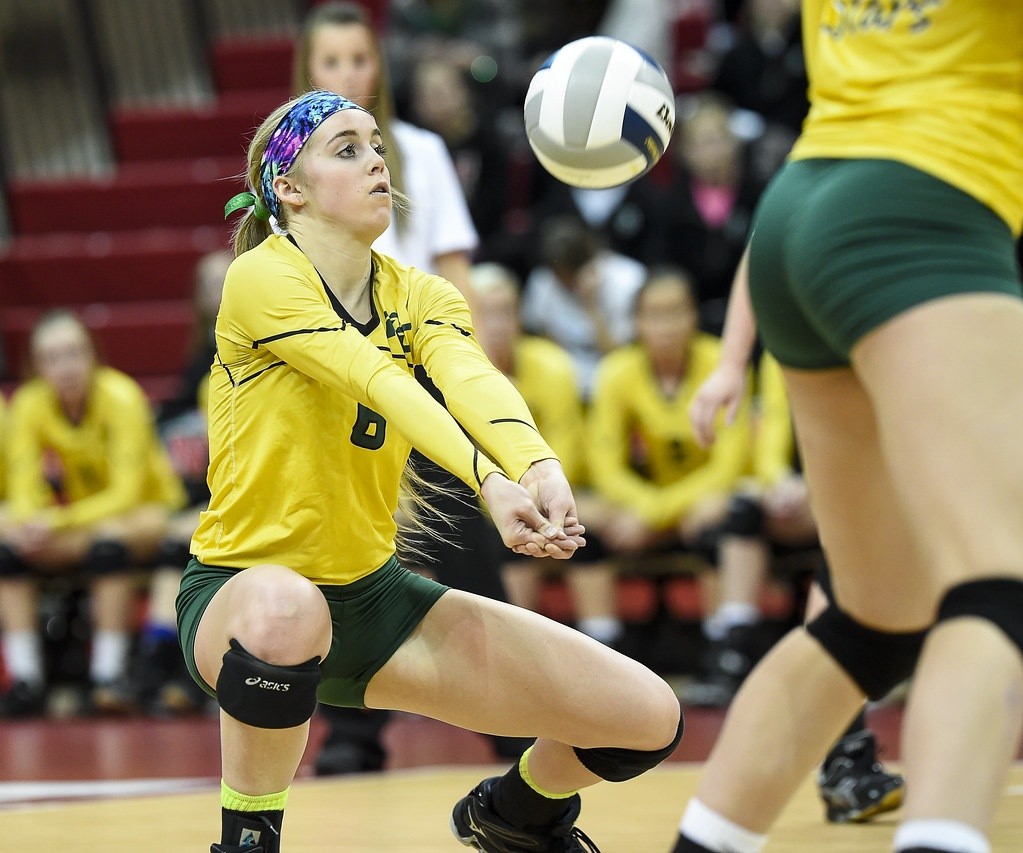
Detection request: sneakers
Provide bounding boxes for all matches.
[449,775,600,853]
[817,732,912,822]
[208,812,281,853]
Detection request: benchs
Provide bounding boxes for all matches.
[0,0,817,628]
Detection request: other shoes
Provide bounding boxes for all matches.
[313,737,389,772]
[0,680,49,724]
[477,730,539,759]
[86,678,131,719]
[682,624,763,706]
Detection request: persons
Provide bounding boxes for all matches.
[667,0,1023,852]
[0,315,191,720]
[166,85,685,852]
[130,0,949,834]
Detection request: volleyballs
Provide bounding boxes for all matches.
[524,35,675,190]
